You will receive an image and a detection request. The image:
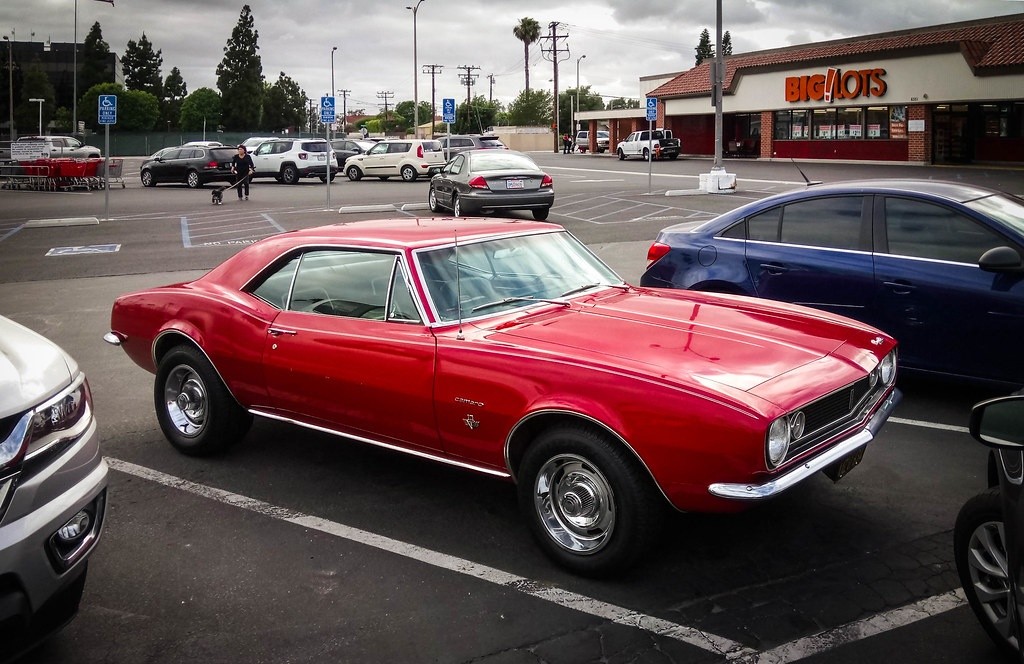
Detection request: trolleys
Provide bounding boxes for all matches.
[3,158,126,192]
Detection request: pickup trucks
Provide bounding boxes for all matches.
[616,130,681,161]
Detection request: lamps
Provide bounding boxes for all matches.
[788,106,888,113]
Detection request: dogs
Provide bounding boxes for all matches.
[212,186,226,205]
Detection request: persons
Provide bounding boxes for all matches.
[231,144,255,201]
[562,133,576,154]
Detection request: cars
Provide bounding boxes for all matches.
[105,214,900,581]
[184,136,404,169]
[0,315,110,663]
[428,148,555,221]
[953,389,1024,663]
[640,178,1024,397]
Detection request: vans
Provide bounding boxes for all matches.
[576,130,609,153]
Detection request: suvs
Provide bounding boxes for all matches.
[342,138,446,182]
[435,134,509,162]
[140,146,243,188]
[249,138,338,184]
[16,136,102,159]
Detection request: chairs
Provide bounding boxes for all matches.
[391,145,399,152]
[451,140,458,146]
[441,277,508,317]
[279,145,285,152]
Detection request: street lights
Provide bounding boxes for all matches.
[576,54,587,132]
[29,98,45,135]
[330,47,338,139]
[406,0,426,138]
[2,36,14,142]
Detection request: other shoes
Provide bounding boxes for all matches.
[245,196,248,200]
[239,197,242,201]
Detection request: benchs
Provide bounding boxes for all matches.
[253,259,410,313]
